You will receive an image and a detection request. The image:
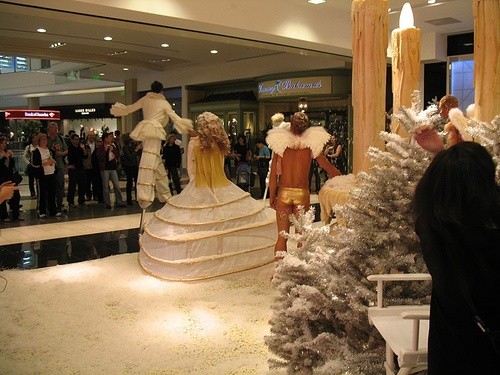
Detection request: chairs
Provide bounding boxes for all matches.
[365,273,433,375]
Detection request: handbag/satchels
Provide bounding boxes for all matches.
[24,164,30,175]
[82,158,92,169]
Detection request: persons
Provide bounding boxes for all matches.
[110,80,193,209]
[0,115,347,220]
[267,111,342,265]
[438,96,463,149]
[409,123,500,375]
[186,111,231,274]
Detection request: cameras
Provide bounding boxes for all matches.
[10,171,23,186]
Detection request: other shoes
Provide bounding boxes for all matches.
[81,203,88,209]
[10,205,24,210]
[106,204,111,209]
[39,214,47,218]
[86,196,90,201]
[4,218,10,222]
[55,212,63,217]
[94,197,98,201]
[16,215,25,220]
[119,203,127,207]
[69,202,76,208]
